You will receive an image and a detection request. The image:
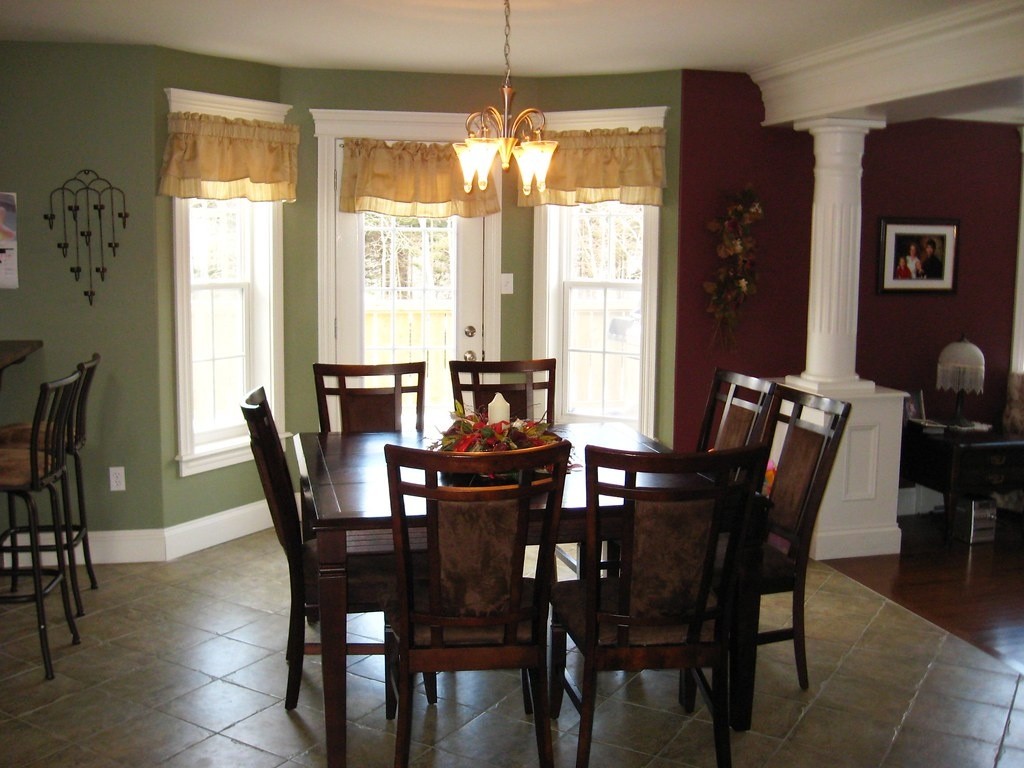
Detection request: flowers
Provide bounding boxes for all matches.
[706,190,763,345]
[430,398,583,487]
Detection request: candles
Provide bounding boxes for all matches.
[488,393,510,424]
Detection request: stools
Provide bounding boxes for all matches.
[0,352,101,679]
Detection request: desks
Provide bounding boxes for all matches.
[300,432,773,768]
[0,340,43,369]
[900,418,1024,552]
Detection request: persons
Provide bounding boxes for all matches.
[895,238,942,279]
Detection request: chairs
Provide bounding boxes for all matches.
[240,358,851,768]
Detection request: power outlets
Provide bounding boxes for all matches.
[109,466,126,491]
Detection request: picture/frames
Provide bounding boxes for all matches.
[902,389,926,426]
[878,216,960,293]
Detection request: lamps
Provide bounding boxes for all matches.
[452,0,559,195]
[937,333,985,426]
